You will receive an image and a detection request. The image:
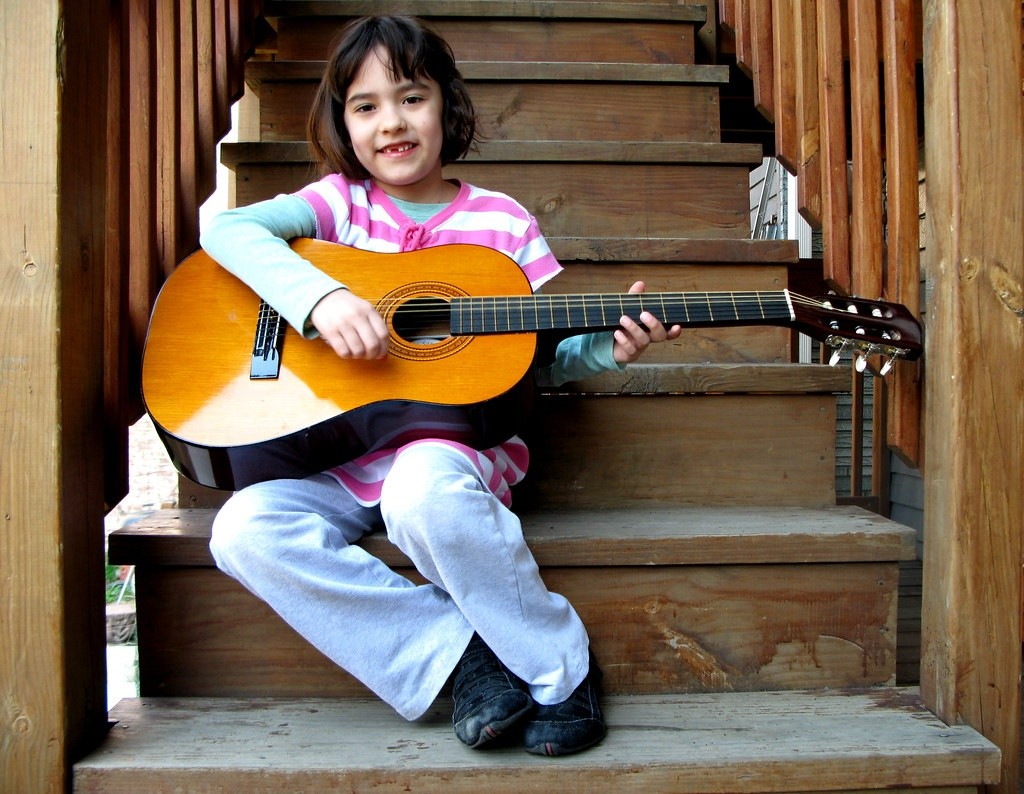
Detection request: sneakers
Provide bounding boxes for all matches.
[524,651,607,756]
[451,635,533,748]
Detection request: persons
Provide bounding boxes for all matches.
[197,17,681,757]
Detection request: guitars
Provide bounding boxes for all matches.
[141,237,923,491]
